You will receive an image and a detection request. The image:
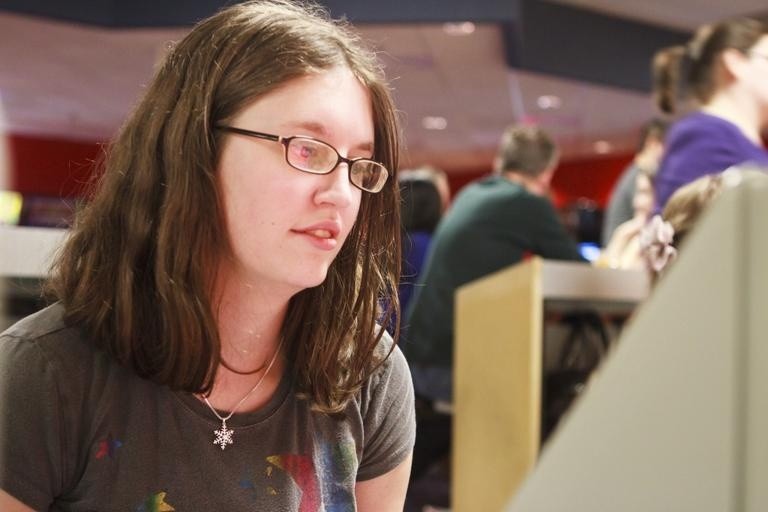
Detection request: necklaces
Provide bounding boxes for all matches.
[196,338,288,452]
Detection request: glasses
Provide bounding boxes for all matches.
[213,125,388,193]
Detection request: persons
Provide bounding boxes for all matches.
[391,123,617,460]
[599,11,768,274]
[0,2,418,512]
